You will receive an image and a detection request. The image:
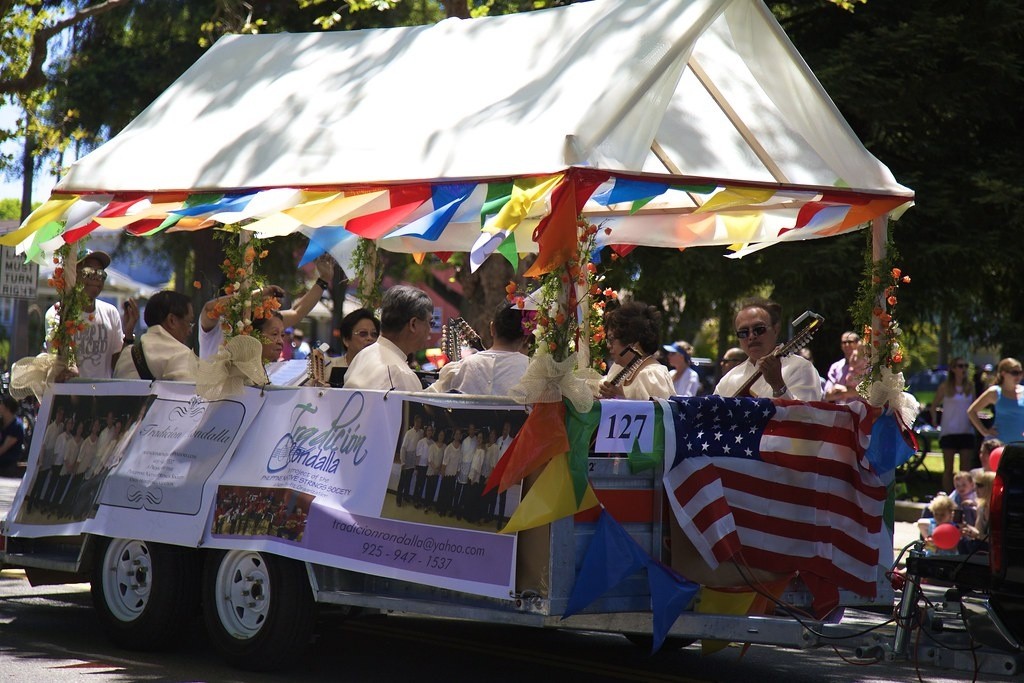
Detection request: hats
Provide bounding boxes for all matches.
[663,343,691,360]
[76,248,111,269]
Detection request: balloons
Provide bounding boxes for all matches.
[989,446,1004,472]
[932,523,960,549]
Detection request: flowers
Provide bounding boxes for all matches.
[503,220,612,374]
[190,220,282,385]
[52,244,98,379]
[851,268,914,391]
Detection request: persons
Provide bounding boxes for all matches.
[930,356,978,496]
[437,301,532,396]
[894,439,1003,581]
[43,248,138,382]
[323,283,435,392]
[195,254,337,362]
[393,414,515,531]
[212,487,308,539]
[713,296,869,401]
[967,358,1024,446]
[0,394,24,479]
[111,290,199,381]
[25,405,146,522]
[599,302,701,402]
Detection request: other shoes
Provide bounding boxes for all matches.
[396,497,502,532]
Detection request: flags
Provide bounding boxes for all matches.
[652,397,881,599]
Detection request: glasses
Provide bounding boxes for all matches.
[720,358,738,364]
[77,267,107,281]
[185,319,195,328]
[350,330,378,339]
[841,340,855,344]
[954,364,969,369]
[407,314,435,328]
[1003,370,1024,376]
[293,335,303,341]
[605,337,620,345]
[735,325,773,339]
[500,442,503,450]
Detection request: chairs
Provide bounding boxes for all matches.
[892,441,1024,654]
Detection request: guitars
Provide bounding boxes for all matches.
[730,319,822,397]
[609,355,637,386]
[449,317,487,353]
[442,319,462,363]
[306,348,325,385]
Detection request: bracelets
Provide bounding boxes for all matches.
[773,385,787,397]
[123,337,135,344]
[316,278,328,290]
